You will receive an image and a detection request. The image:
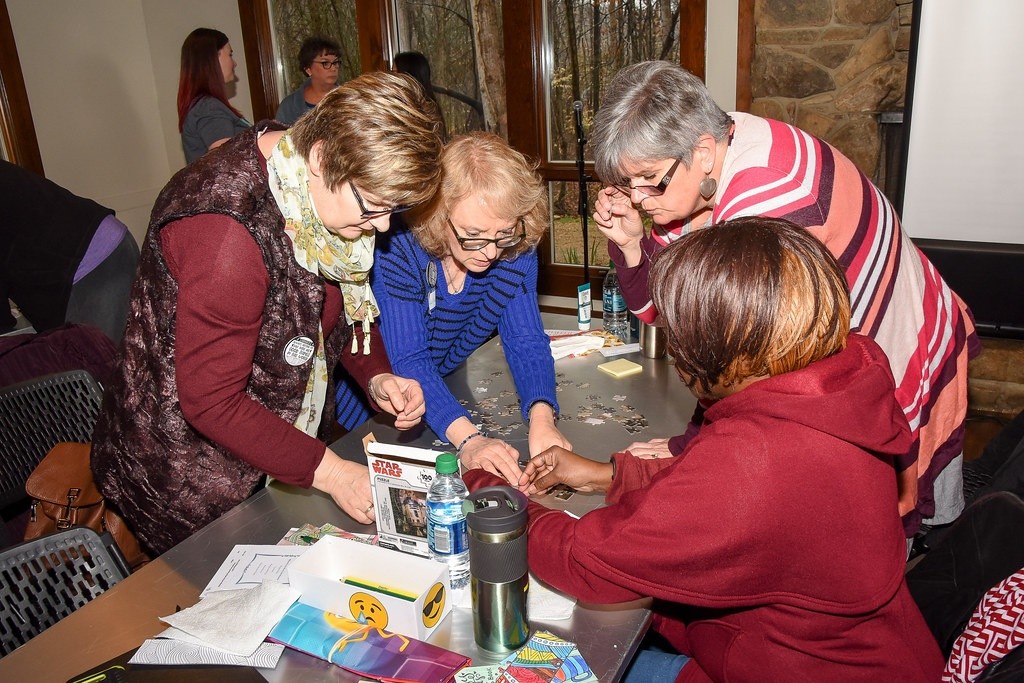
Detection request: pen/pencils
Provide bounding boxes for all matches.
[339,576,418,602]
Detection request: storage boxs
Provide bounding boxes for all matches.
[361,432,462,559]
[286,533,451,644]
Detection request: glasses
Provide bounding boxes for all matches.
[343,170,413,218]
[313,60,341,70]
[444,215,526,251]
[613,150,684,198]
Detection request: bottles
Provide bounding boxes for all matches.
[602,258,628,344]
[425,453,473,589]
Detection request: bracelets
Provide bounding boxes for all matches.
[368,381,375,401]
[457,432,482,451]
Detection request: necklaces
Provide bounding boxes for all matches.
[444,260,466,293]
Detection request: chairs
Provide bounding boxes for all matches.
[0,525,134,658]
[904,490,1024,683]
[0,368,106,510]
[917,409,1024,534]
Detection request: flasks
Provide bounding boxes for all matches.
[461,486,529,662]
[639,315,670,357]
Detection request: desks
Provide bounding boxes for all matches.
[0,313,698,683]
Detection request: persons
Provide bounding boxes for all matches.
[462,216,947,683]
[331,131,574,487]
[274,38,343,124]
[90,72,442,563]
[0,159,142,347]
[392,52,436,102]
[177,28,251,165]
[590,59,981,563]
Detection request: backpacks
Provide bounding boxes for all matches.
[23,442,150,593]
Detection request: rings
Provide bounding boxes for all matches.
[652,454,657,459]
[365,504,374,514]
